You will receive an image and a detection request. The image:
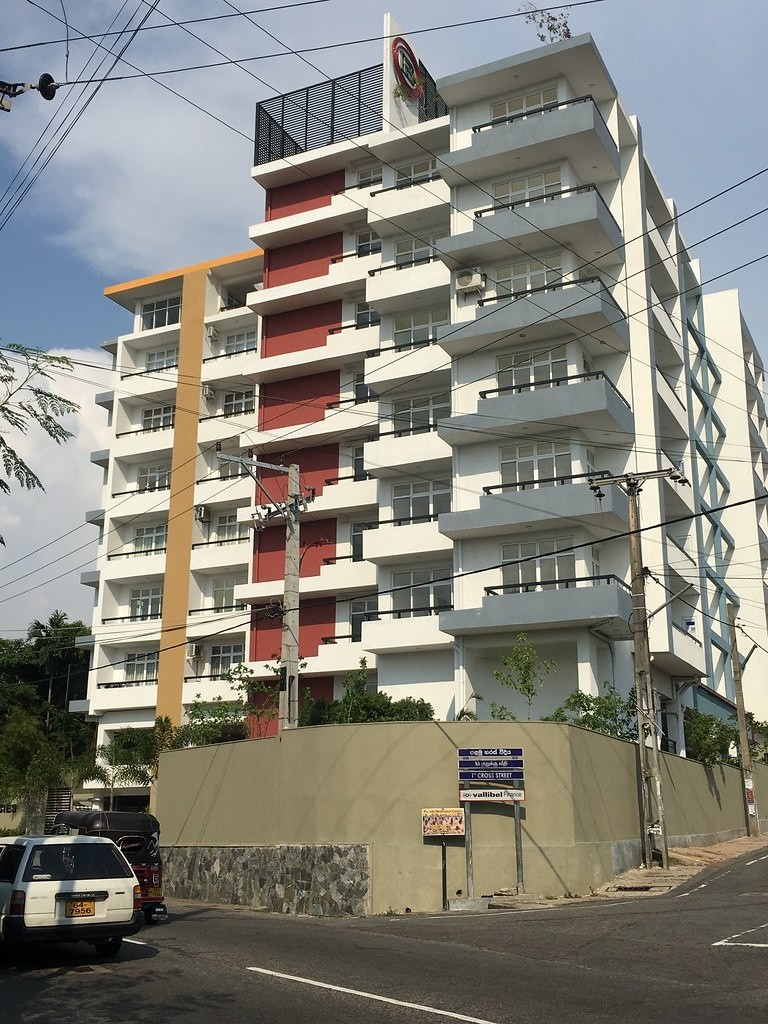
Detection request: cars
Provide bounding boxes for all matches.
[0,835,142,960]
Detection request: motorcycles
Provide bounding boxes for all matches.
[52,811,168,925]
